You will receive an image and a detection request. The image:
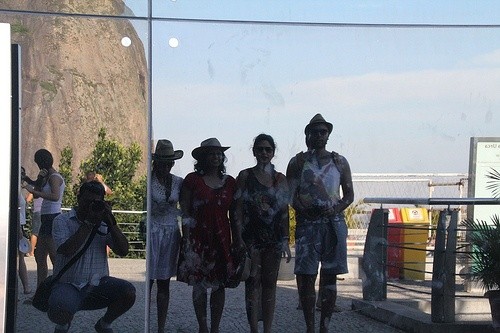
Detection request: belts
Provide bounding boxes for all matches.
[300,216,343,223]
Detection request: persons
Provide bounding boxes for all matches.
[17,149,112,300]
[48,181,136,333]
[149,140,183,333]
[229,134,291,333]
[177,138,241,333]
[286,114,354,333]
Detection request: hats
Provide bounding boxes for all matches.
[150,140,183,160]
[192,138,230,161]
[305,114,333,136]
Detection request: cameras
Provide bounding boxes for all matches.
[307,206,321,217]
[89,201,106,212]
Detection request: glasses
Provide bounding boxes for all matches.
[256,147,273,153]
[158,161,174,167]
[309,129,327,135]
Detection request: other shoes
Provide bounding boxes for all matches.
[24,294,35,304]
[55,322,70,333]
[95,317,113,333]
[317,297,343,312]
[24,289,32,294]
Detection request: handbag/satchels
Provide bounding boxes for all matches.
[19,237,32,255]
[32,276,53,312]
[236,247,253,281]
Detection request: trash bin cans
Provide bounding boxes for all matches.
[372,208,401,278]
[401,208,431,280]
